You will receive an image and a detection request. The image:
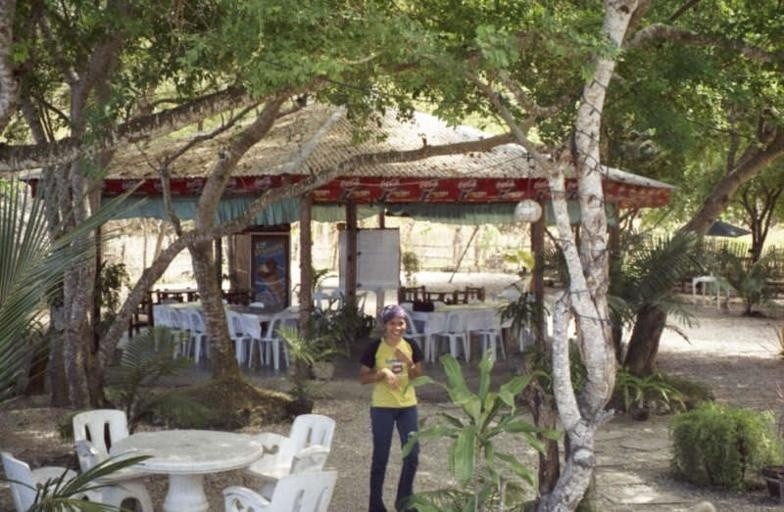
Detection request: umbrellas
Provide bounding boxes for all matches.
[706,220,749,237]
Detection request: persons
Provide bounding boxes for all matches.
[357,303,424,512]
[258,256,286,300]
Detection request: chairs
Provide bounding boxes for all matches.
[246,414,336,483]
[0,453,80,512]
[72,407,155,511]
[222,469,339,512]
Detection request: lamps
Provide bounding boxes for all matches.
[512,152,543,224]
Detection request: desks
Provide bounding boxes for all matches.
[692,275,732,313]
[110,430,265,512]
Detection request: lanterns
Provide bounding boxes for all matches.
[512,197,542,223]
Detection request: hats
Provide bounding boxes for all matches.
[383,305,406,324]
